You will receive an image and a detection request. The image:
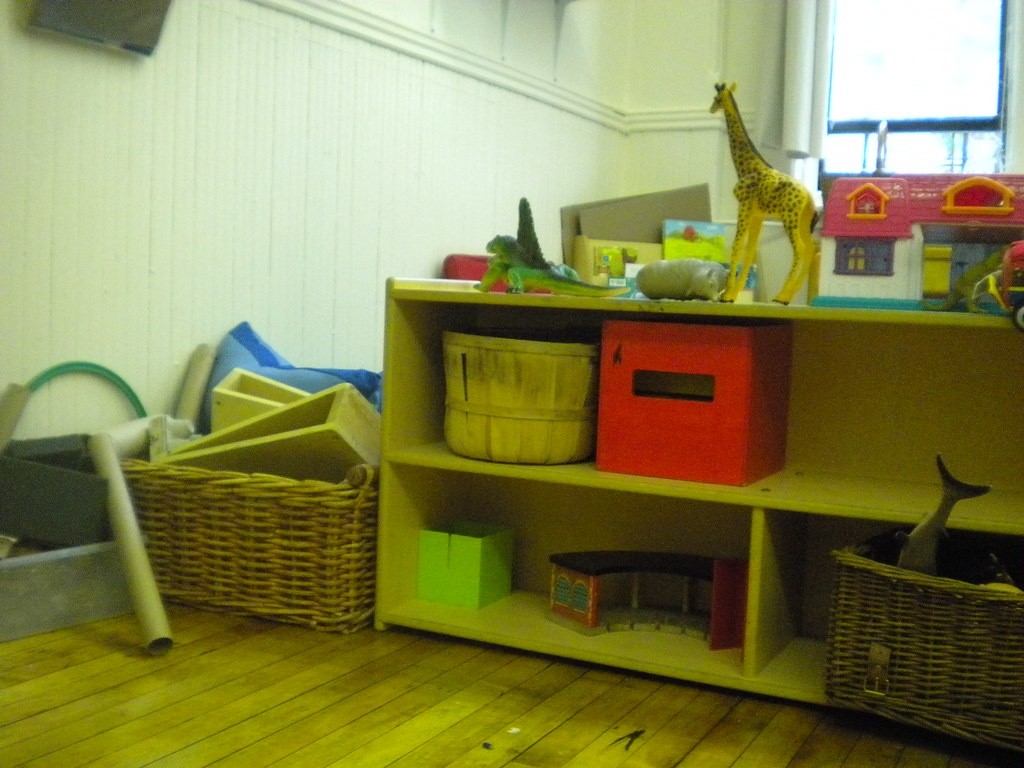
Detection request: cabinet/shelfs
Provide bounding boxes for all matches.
[373,278,1024,705]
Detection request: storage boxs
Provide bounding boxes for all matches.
[109,455,378,636]
[0,434,147,643]
[828,528,1023,754]
[416,520,517,613]
[591,317,794,486]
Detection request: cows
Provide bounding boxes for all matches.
[637,259,727,303]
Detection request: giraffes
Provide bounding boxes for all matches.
[709,81,816,304]
